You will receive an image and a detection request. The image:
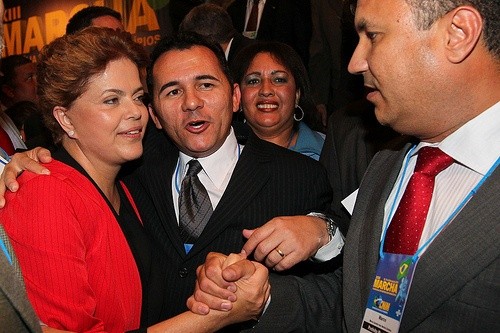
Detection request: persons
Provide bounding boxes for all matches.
[65,7,122,31]
[0,26,271,333]
[0,28,352,333]
[343,0,500,333]
[1,54,39,159]
[180,6,243,61]
[280,0,393,201]
[232,37,326,162]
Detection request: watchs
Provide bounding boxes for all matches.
[318,216,337,241]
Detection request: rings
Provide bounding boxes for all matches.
[275,247,285,257]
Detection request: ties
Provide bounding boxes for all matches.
[246,0,259,31]
[178,160,213,244]
[383,146,455,255]
